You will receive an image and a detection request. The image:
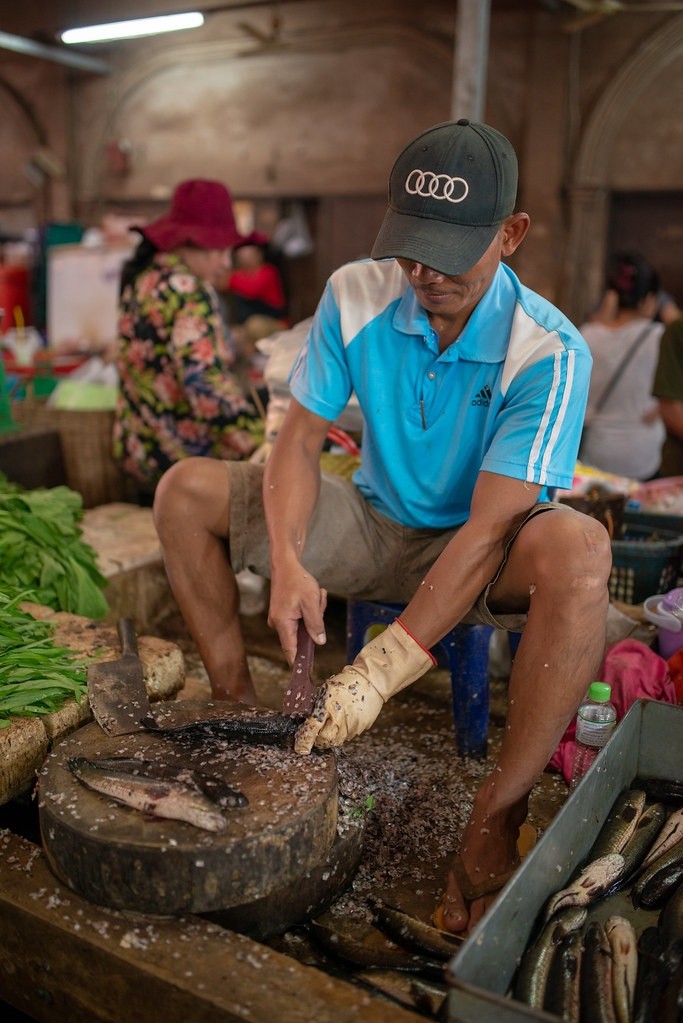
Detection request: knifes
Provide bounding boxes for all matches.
[84,617,153,740]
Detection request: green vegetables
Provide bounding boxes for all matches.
[0,473,109,730]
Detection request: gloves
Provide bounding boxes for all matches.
[294,616,438,755]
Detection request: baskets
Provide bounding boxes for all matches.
[9,396,138,511]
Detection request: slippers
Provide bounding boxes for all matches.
[431,822,539,943]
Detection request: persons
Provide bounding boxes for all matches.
[642,316,683,487]
[108,177,268,487]
[224,239,291,321]
[577,263,669,485]
[150,116,615,935]
[586,248,681,333]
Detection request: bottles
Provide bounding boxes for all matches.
[566,681,618,803]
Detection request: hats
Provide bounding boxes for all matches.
[371,118,519,274]
[128,177,268,252]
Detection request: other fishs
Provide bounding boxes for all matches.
[305,899,469,1019]
[512,773,683,1023]
[68,717,299,833]
